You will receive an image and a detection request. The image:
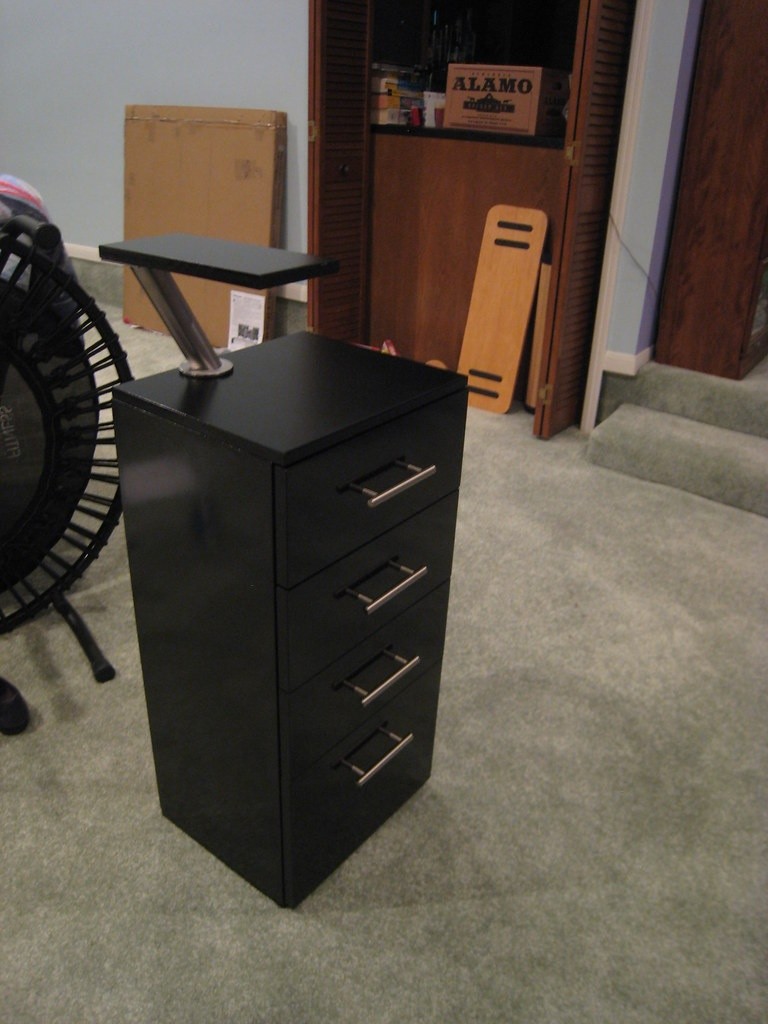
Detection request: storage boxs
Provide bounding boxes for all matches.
[442,62,568,135]
[124,103,288,349]
[372,64,398,92]
[371,94,401,123]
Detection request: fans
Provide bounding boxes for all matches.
[0,214,135,682]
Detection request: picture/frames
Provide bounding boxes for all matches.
[422,91,447,127]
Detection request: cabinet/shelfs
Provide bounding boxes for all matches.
[113,329,467,907]
[656,0,768,381]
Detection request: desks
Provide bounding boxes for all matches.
[99,232,339,373]
